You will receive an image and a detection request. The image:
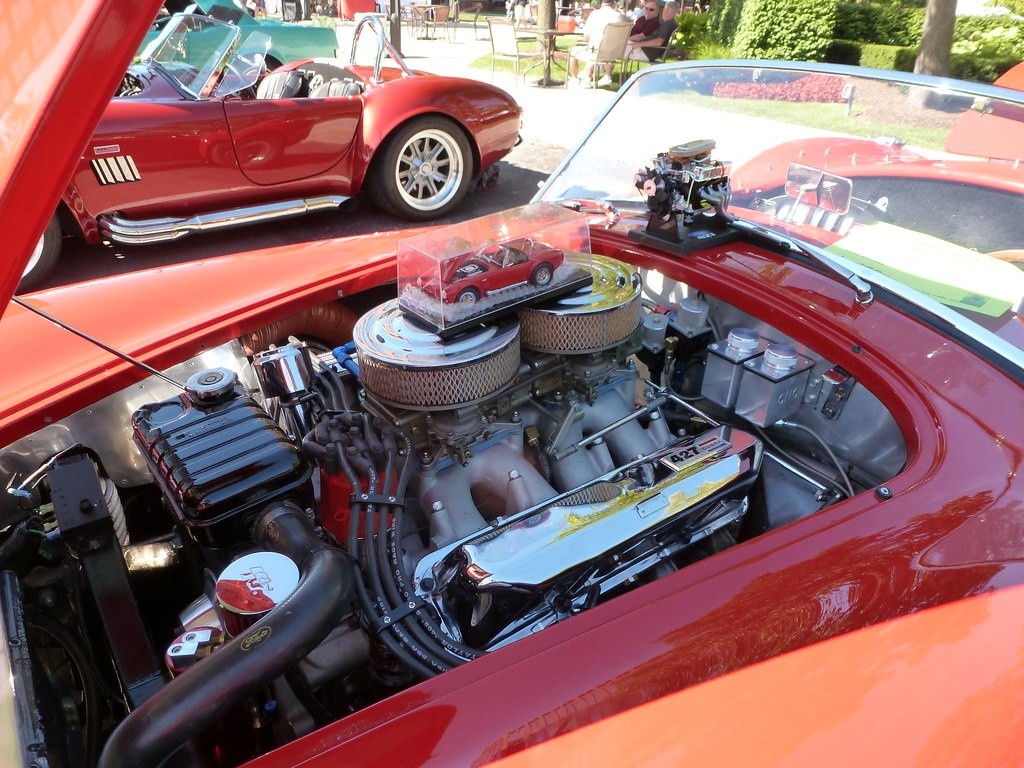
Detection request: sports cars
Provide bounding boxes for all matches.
[1,0,1024,768]
[113,0,338,99]
[14,13,524,283]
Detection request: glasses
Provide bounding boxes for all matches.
[645,7,654,11]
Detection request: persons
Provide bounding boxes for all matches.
[567,0,622,90]
[311,2,338,17]
[249,0,267,19]
[580,0,679,87]
[505,0,518,20]
[513,0,528,29]
[694,0,701,15]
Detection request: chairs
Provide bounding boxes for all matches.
[256,71,303,100]
[489,17,535,81]
[568,23,634,86]
[422,6,451,43]
[626,26,684,80]
[454,4,482,37]
[308,79,361,98]
[384,4,416,38]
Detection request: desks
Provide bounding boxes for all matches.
[516,28,572,87]
[412,6,436,40]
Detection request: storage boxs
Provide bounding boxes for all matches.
[398,200,594,335]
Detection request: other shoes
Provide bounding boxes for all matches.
[591,75,612,87]
[581,78,592,87]
[567,78,579,88]
